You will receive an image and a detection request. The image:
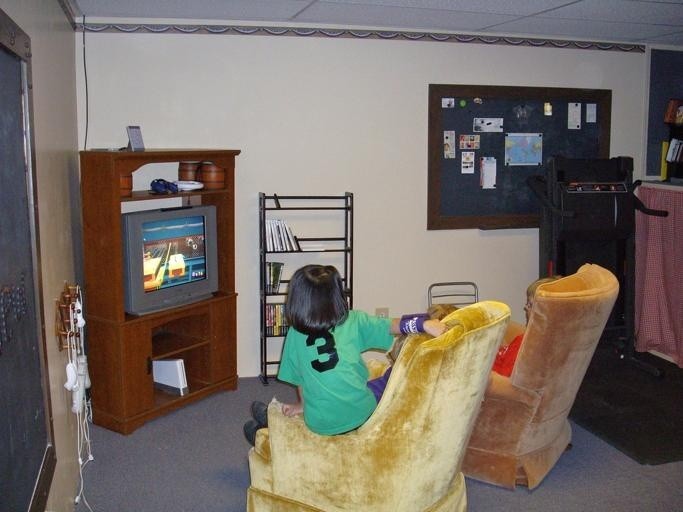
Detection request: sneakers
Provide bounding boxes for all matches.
[243,420,255,445]
[251,400,267,425]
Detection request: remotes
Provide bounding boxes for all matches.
[160,206,192,212]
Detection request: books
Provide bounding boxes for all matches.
[263,218,293,338]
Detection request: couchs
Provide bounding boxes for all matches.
[463,263,620,492]
[246,300,511,508]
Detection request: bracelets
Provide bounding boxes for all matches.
[398,316,429,336]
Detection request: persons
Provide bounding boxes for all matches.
[491,276,559,377]
[241,302,462,449]
[275,263,449,434]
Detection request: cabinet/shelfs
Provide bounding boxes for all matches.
[256,191,354,387]
[76,145,242,436]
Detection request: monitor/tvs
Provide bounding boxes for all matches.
[121,204,219,318]
